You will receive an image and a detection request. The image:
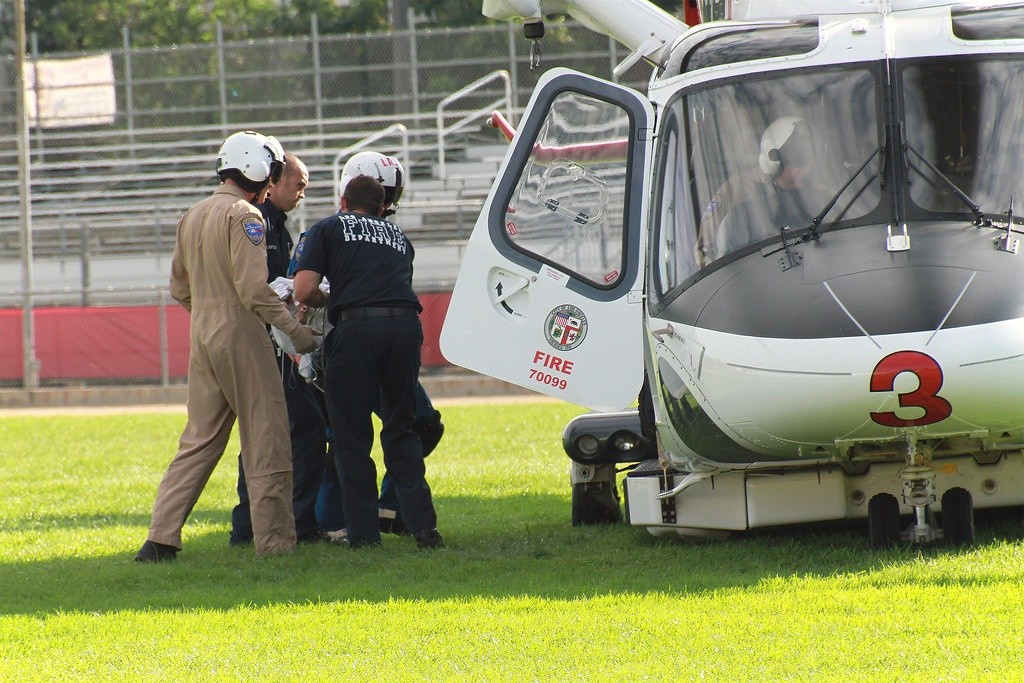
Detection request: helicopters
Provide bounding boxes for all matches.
[436,0,1023,548]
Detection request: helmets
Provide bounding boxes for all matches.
[758,116,823,176]
[340,151,407,219]
[216,131,287,193]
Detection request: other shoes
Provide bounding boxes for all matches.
[229,540,246,546]
[135,540,177,563]
[319,527,355,548]
[414,529,444,550]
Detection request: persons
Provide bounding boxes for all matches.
[692,117,855,270]
[134,130,324,562]
[286,175,445,551]
[313,151,445,544]
[228,151,348,549]
